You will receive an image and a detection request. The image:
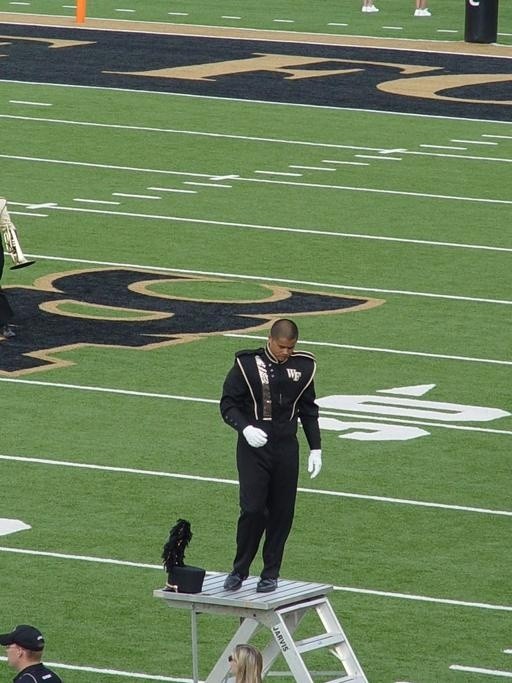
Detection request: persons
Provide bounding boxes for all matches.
[223,644,262,683]
[362,0,379,12]
[219,319,322,595]
[0,624,63,683]
[0,231,16,340]
[414,0,431,16]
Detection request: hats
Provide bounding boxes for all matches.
[2,624,44,652]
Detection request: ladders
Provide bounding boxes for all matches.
[153,570,369,683]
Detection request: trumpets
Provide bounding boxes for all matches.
[0,198,36,270]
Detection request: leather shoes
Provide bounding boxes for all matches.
[256,574,279,593]
[223,567,249,592]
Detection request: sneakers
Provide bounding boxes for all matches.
[413,8,433,17]
[362,4,380,13]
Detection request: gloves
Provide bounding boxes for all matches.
[239,424,270,449]
[307,449,324,480]
